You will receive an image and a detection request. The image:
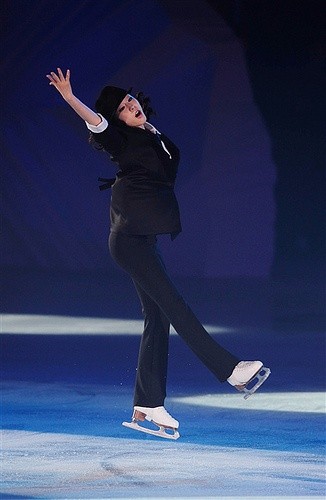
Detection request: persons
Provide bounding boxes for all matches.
[47,69,274,441]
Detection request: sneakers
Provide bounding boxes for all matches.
[121,404,181,441]
[227,360,270,400]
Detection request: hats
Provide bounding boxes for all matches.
[96,84,132,122]
